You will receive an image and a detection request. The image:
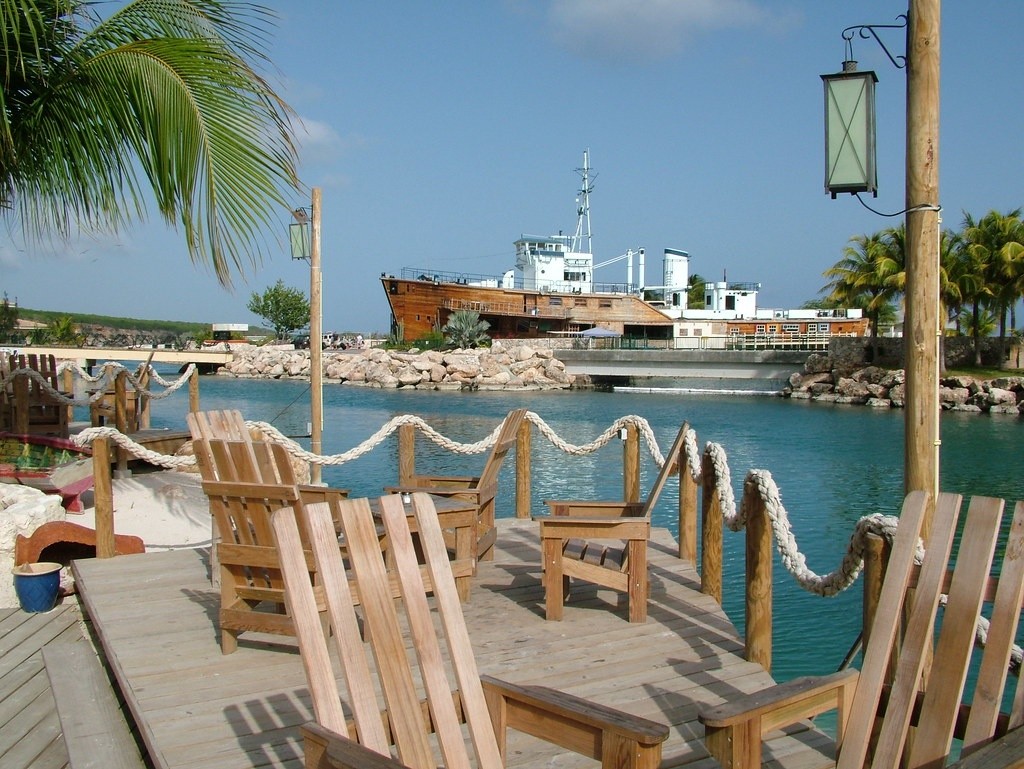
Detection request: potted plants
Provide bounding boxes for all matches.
[12,561,63,613]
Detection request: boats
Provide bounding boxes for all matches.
[0,430,113,515]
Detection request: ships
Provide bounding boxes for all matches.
[380,145,870,350]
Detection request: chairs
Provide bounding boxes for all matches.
[9,353,74,439]
[268,493,670,769]
[192,438,392,654]
[697,491,1024,769]
[85,350,156,434]
[186,409,351,589]
[383,408,528,561]
[532,420,690,623]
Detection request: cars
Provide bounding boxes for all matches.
[323,333,341,347]
[290,334,327,351]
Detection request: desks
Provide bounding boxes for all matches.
[360,493,480,604]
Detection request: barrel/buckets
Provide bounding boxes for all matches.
[11,561,63,613]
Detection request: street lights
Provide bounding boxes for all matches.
[289,187,323,488]
[818,0,942,769]
